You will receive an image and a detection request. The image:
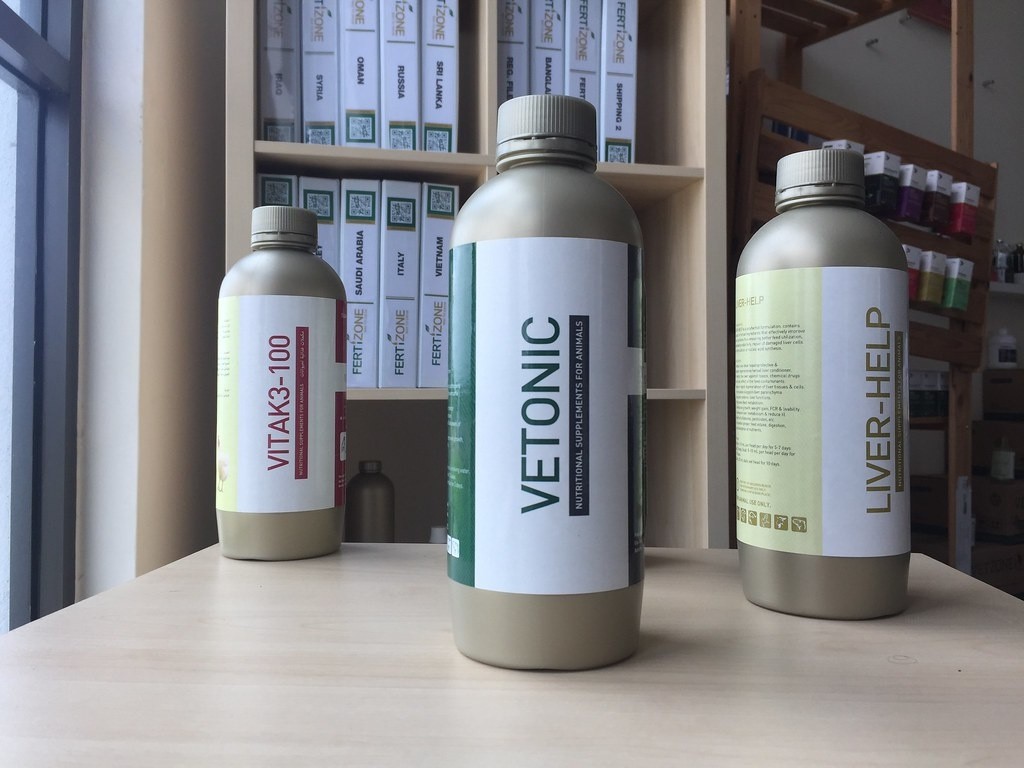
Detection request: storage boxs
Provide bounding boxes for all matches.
[923,171,953,218]
[498,1,639,161]
[918,248,945,304]
[822,140,865,156]
[972,420,1024,473]
[910,430,945,476]
[864,150,901,212]
[944,258,974,311]
[904,245,922,299]
[908,355,949,391]
[256,0,460,153]
[990,452,1015,479]
[910,480,1024,534]
[257,174,457,389]
[896,164,927,219]
[983,369,1024,414]
[971,544,1024,595]
[946,183,980,236]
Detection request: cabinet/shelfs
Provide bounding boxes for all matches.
[223,0,732,547]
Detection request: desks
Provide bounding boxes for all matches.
[0,543,1024,768]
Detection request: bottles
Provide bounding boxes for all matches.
[989,437,1016,483]
[991,240,1024,284]
[446,94,644,671]
[215,205,348,559]
[735,148,912,620]
[993,329,1020,368]
